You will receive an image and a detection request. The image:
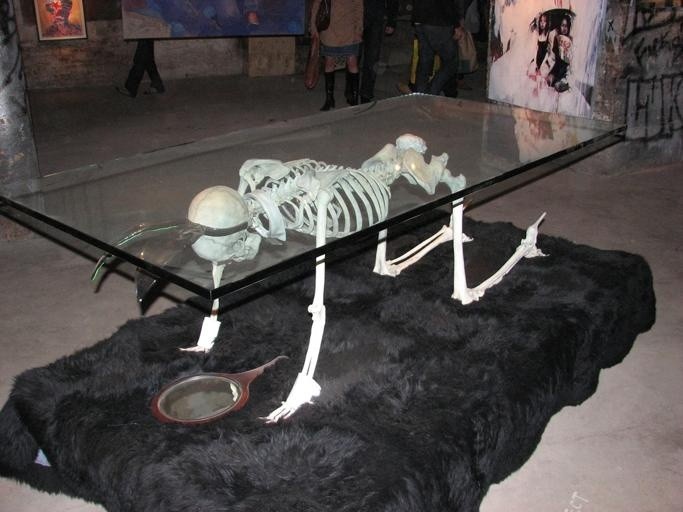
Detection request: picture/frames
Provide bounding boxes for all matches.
[34,0,88,42]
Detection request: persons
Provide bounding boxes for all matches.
[115,39,165,98]
[308,0,480,110]
[178,133,550,425]
[529,8,576,115]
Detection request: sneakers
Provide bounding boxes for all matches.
[459,81,472,91]
[115,85,159,98]
[396,83,412,95]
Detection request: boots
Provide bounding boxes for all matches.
[348,72,358,106]
[320,71,335,111]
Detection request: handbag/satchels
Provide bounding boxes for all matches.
[453,27,477,72]
[315,0,330,31]
[304,38,319,89]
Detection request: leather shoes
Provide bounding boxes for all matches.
[361,93,373,103]
